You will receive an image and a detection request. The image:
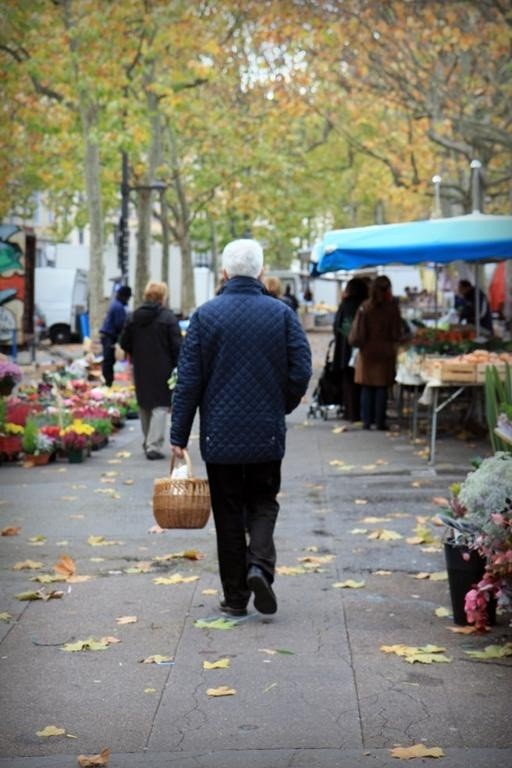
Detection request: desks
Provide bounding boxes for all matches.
[397,353,512,466]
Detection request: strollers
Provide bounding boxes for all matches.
[308,339,348,419]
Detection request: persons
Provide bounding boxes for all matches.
[459,279,493,336]
[283,283,298,311]
[262,275,294,311]
[333,277,368,423]
[348,276,401,430]
[168,239,312,617]
[98,286,131,388]
[118,282,183,461]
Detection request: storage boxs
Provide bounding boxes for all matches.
[423,358,474,384]
[474,362,512,383]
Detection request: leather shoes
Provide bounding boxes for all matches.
[246,565,277,614]
[218,601,247,616]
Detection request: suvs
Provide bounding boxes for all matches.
[0,302,47,354]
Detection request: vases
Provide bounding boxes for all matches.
[443,543,496,626]
[33,454,48,464]
[67,449,82,463]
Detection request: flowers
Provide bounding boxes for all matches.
[0,358,139,456]
[438,483,489,545]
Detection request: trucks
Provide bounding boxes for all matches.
[33,268,90,344]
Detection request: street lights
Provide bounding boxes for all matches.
[467,159,486,339]
[429,171,445,218]
[120,180,168,282]
